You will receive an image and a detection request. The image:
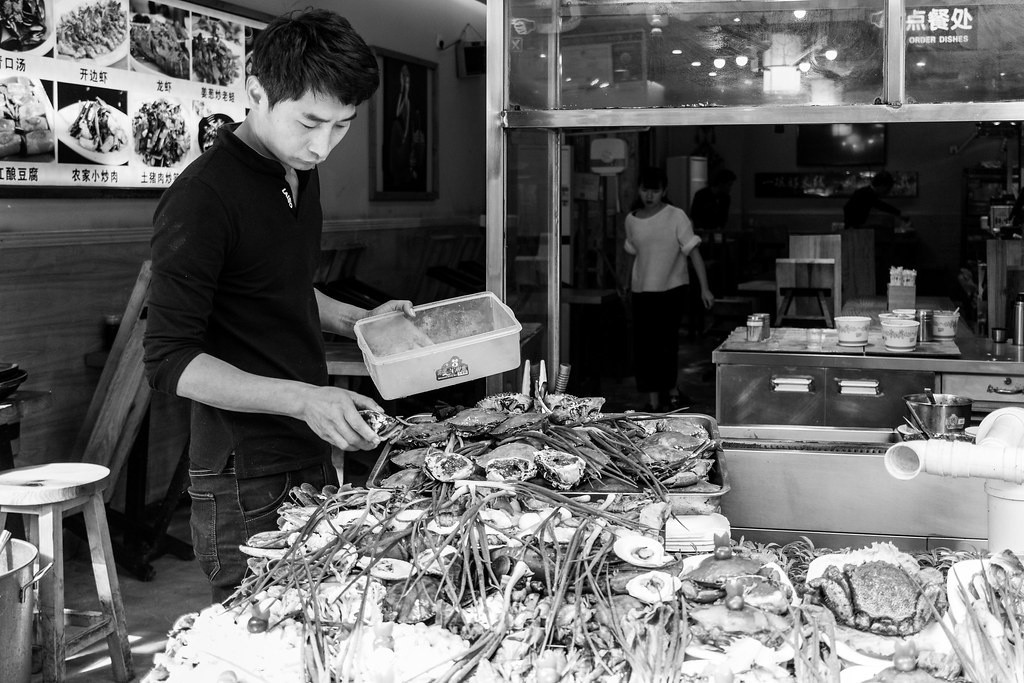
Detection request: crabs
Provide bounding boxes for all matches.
[275,391,715,630]
[683,550,1024,637]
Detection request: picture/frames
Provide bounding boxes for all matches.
[364,45,441,202]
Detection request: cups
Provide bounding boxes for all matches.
[747,312,771,342]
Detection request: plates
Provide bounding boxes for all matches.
[58,101,131,166]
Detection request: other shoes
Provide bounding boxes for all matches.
[668,389,679,408]
[644,402,662,412]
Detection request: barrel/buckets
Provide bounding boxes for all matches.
[985,479,1024,559]
[0,538,54,683]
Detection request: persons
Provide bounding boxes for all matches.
[844,171,898,280]
[142,10,431,606]
[623,168,736,411]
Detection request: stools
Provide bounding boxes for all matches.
[0,460,135,683]
[776,286,833,328]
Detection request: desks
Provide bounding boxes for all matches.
[514,285,617,364]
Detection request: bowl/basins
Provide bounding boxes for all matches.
[879,309,961,352]
[834,316,871,347]
[354,291,522,400]
[903,392,974,437]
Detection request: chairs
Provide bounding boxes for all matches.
[511,300,573,366]
